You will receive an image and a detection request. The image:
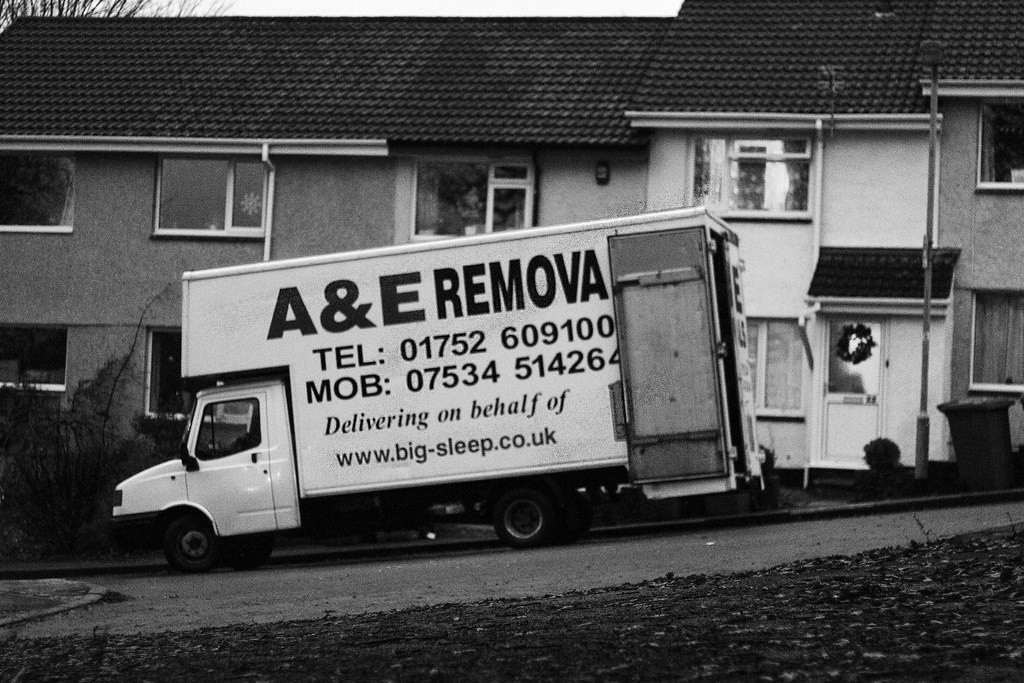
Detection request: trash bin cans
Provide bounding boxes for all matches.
[938,396,1019,493]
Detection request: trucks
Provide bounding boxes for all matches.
[104,206,766,575]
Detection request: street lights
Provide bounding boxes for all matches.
[914,35,948,473]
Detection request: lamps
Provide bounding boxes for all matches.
[595,159,613,185]
